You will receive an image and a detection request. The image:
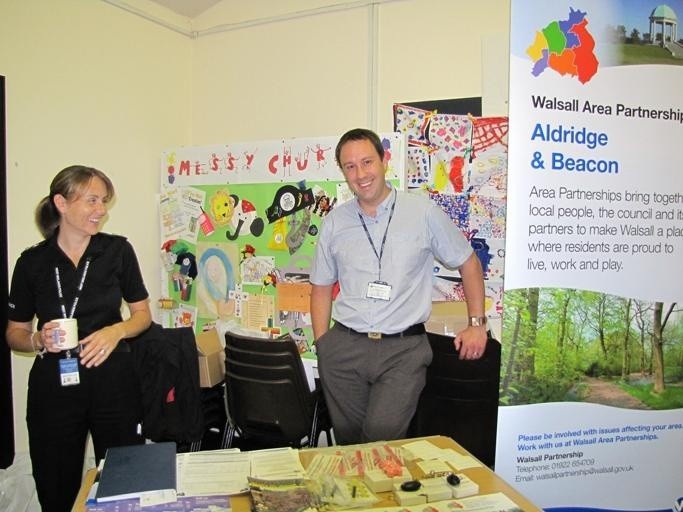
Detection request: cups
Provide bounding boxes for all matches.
[50,318,79,351]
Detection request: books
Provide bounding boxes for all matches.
[95,441,178,503]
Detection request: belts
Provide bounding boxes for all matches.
[335,321,425,340]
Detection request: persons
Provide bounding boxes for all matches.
[3,165,153,510]
[305,127,488,446]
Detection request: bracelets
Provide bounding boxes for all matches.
[467,316,486,328]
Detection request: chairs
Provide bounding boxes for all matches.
[127,321,332,453]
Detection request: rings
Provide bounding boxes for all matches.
[473,351,479,356]
[99,349,105,355]
